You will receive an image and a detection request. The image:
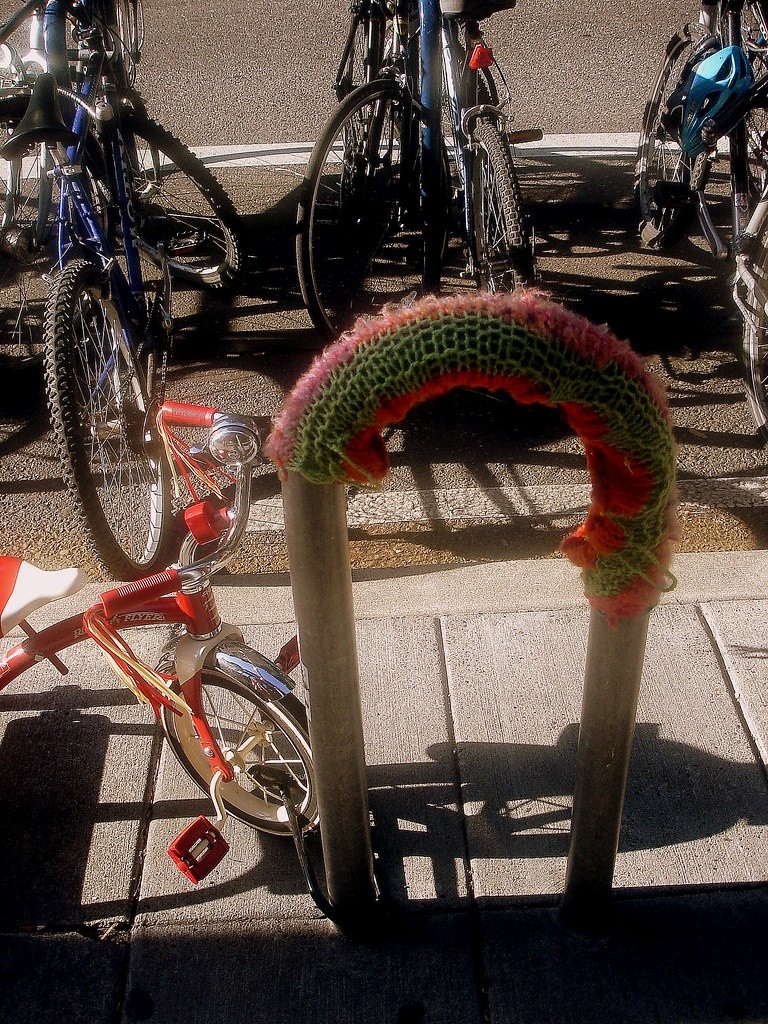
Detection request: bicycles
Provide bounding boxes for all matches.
[0,0,252,583]
[288,1,546,348]
[635,1,768,450]
[0,399,325,889]
